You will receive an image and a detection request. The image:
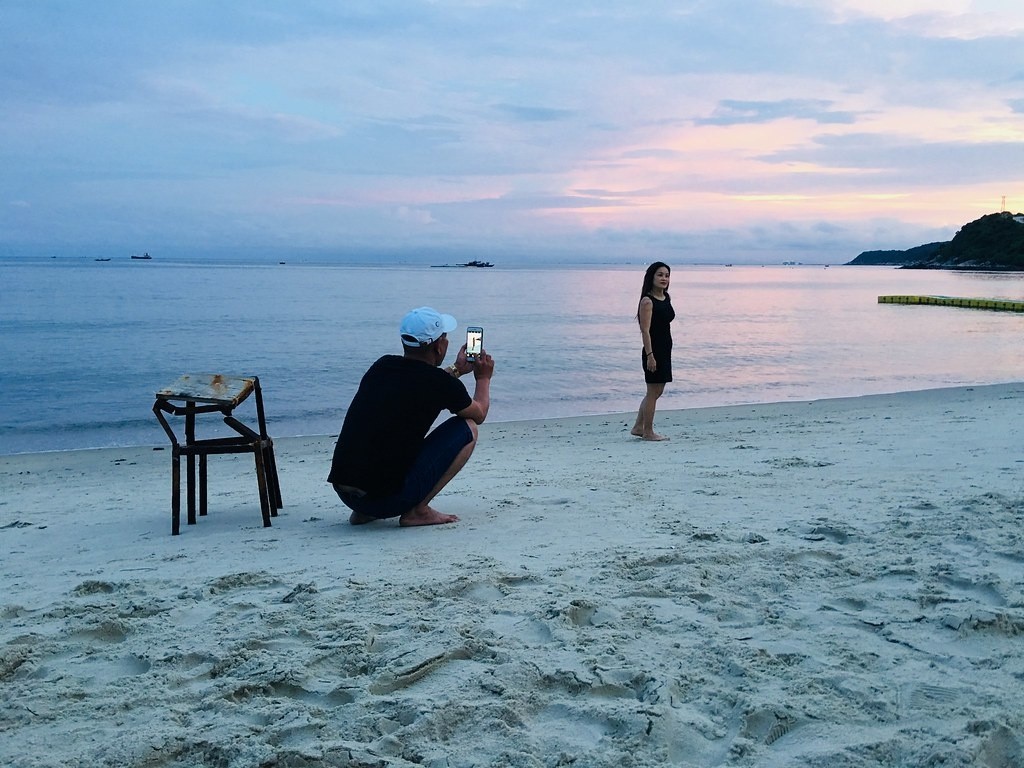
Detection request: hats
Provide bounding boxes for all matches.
[399,306,457,347]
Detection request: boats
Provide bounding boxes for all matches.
[466,260,494,267]
[95,258,110,261]
[131,253,152,259]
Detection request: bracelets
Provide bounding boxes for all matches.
[448,364,461,379]
[647,352,653,356]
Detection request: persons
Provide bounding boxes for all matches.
[327,307,494,528]
[471,337,475,350]
[631,261,676,441]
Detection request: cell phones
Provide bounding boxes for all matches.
[466,327,483,363]
